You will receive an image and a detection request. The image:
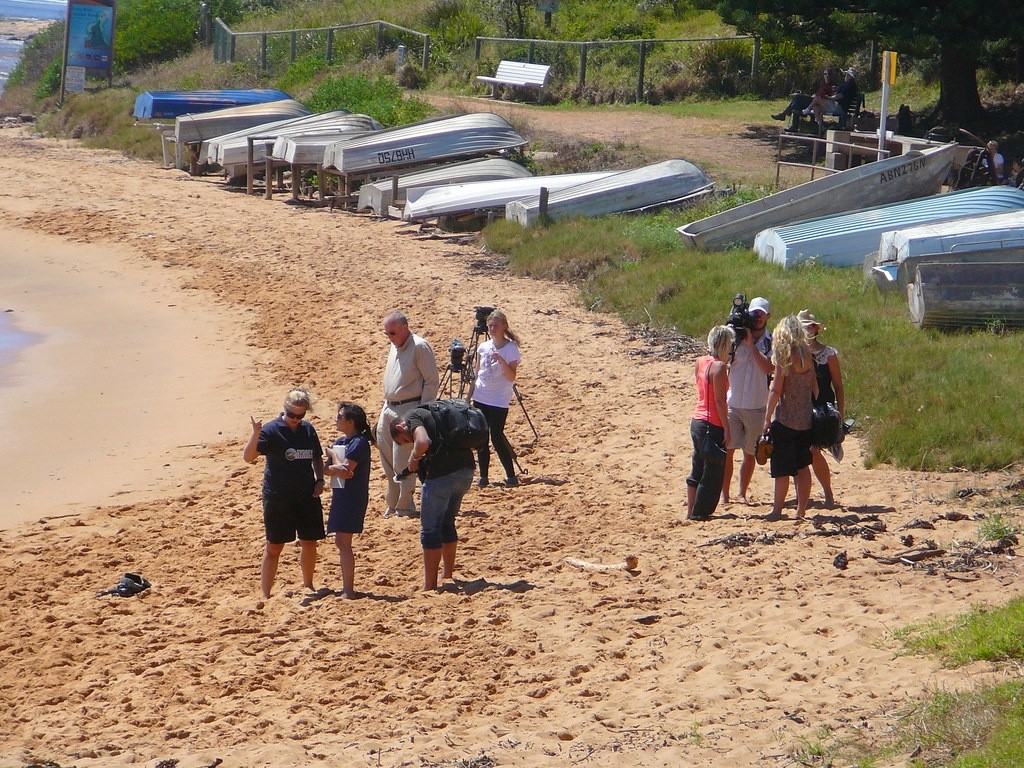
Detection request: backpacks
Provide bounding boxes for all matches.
[417,399,488,450]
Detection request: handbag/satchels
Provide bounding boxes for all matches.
[856,112,875,131]
[700,433,727,466]
[812,399,845,446]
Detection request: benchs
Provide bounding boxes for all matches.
[803,93,864,137]
[477,60,550,104]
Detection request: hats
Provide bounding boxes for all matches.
[748,296,769,315]
[844,67,857,78]
[797,309,823,330]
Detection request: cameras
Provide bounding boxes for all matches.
[392,454,429,485]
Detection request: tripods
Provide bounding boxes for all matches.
[438,326,538,474]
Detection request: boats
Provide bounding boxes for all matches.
[676,140,959,254]
[175,99,385,177]
[403,170,627,220]
[133,89,296,119]
[874,210,1024,328]
[506,159,716,230]
[753,185,1024,270]
[272,130,379,164]
[322,111,530,172]
[357,157,533,218]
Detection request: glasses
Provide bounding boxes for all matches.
[338,414,347,420]
[285,406,305,420]
[384,326,404,335]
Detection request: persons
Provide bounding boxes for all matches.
[795,308,844,500]
[686,325,736,516]
[982,140,1004,185]
[763,314,819,518]
[243,391,325,598]
[389,401,476,591]
[792,67,856,134]
[376,311,439,516]
[770,68,838,132]
[1002,157,1024,190]
[323,402,371,600]
[723,296,771,504]
[467,310,521,487]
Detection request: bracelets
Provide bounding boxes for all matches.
[316,479,325,485]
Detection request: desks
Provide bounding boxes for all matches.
[849,132,985,177]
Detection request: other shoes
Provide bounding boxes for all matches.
[771,113,786,121]
[784,126,798,132]
[765,433,773,458]
[477,476,489,488]
[792,108,807,118]
[395,509,411,515]
[505,476,518,487]
[383,507,396,519]
[754,435,767,465]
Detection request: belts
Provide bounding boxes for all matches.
[387,396,421,405]
